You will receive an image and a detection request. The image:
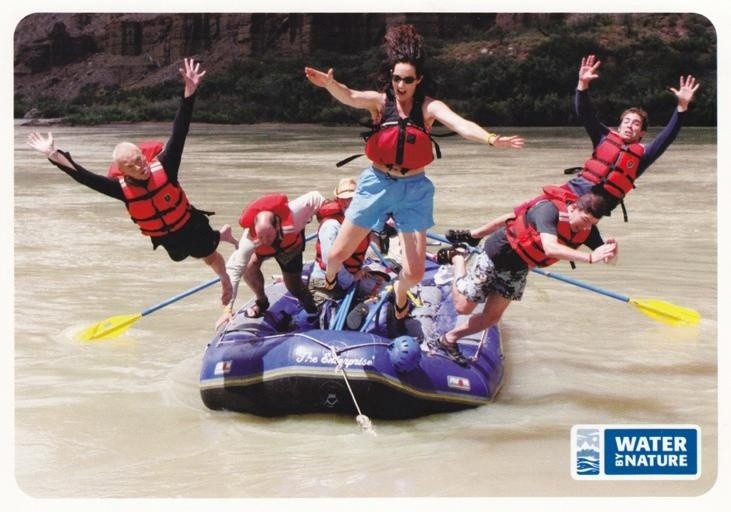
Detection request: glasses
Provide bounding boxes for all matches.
[120,154,144,172]
[391,75,417,83]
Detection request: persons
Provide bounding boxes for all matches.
[431,193,620,368]
[302,23,527,322]
[310,176,397,330]
[215,190,325,330]
[444,52,703,249]
[24,57,240,306]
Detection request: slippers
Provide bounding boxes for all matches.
[393,299,410,321]
[325,273,338,291]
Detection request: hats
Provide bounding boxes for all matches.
[333,177,357,199]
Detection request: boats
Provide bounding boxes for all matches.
[203,255,504,421]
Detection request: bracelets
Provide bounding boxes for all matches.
[589,253,594,264]
[222,308,233,313]
[47,149,57,159]
[493,135,502,146]
[487,132,496,145]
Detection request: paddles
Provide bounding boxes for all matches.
[427,231,700,328]
[84,233,317,342]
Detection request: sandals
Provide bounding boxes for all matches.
[437,228,481,265]
[244,298,270,318]
[435,334,468,367]
